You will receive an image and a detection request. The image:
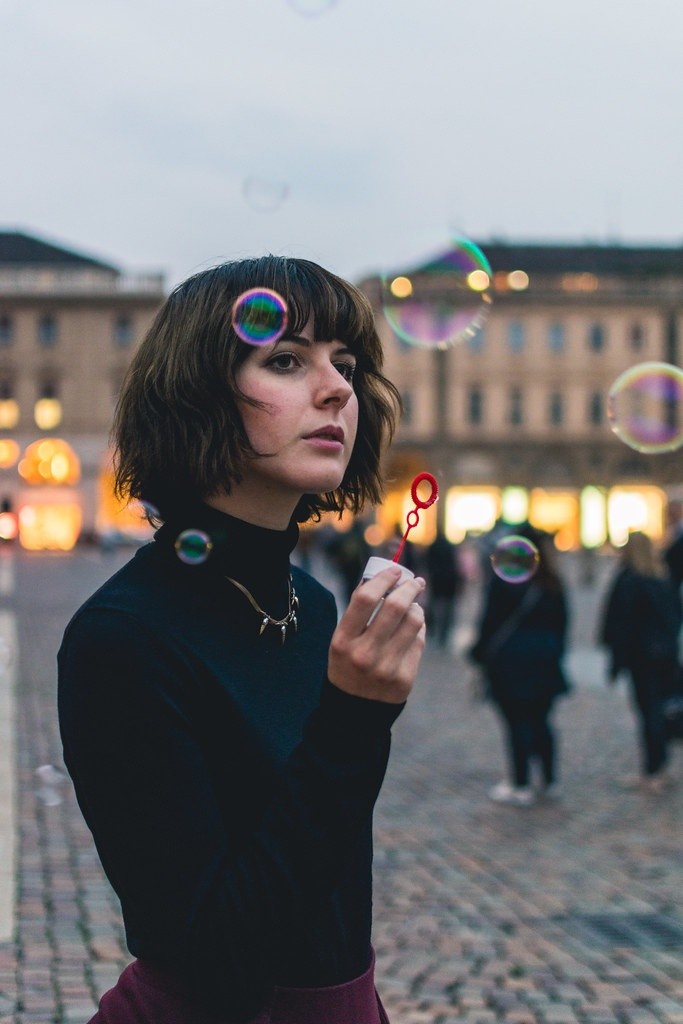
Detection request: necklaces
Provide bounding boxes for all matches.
[225,575,299,645]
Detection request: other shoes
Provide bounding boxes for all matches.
[488,784,535,806]
[532,782,565,800]
[623,771,670,795]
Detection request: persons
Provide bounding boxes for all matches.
[58,256,427,1024]
[600,531,683,793]
[465,525,569,806]
[296,519,464,649]
